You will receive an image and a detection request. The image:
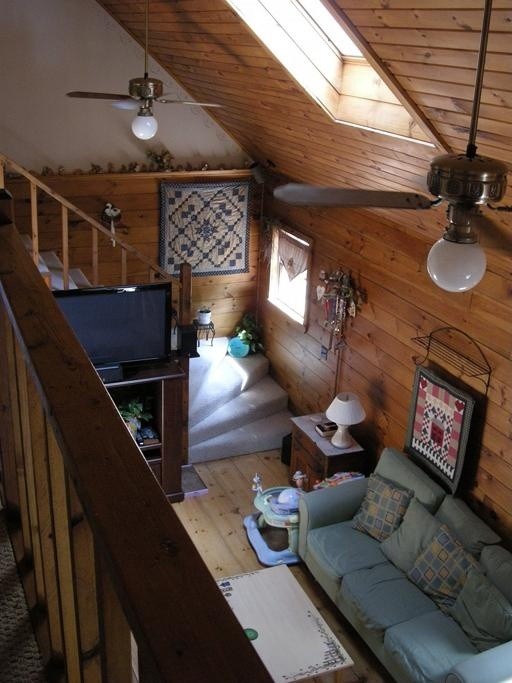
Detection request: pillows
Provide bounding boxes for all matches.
[348,468,512,655]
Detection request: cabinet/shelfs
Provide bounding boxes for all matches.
[97,362,189,506]
[287,411,365,497]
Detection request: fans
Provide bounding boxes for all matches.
[66,0,232,116]
[269,1,511,221]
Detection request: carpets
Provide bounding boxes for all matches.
[221,564,356,680]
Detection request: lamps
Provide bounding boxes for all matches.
[324,388,366,448]
[129,102,161,140]
[425,196,491,296]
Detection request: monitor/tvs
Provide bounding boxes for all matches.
[51,280,173,373]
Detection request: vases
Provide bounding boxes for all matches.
[198,311,212,324]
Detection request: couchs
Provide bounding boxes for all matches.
[297,443,512,683]
